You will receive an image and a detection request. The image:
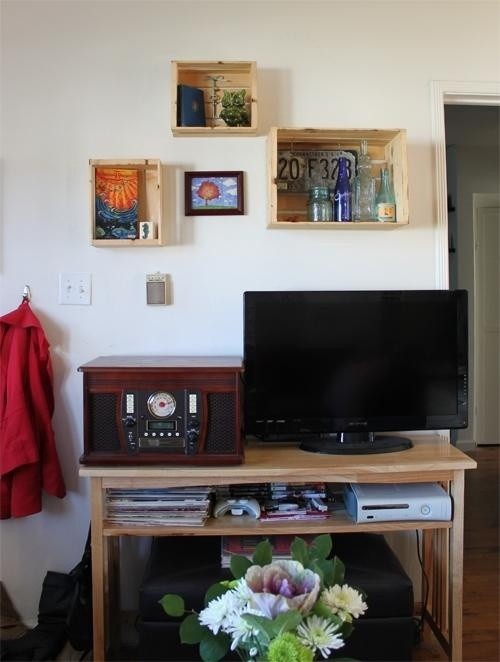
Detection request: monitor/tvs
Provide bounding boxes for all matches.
[242,289,470,454]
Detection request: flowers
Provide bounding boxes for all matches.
[155,535,370,662]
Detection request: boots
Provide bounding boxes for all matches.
[0,568,69,661]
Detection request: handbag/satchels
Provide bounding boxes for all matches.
[66,524,95,651]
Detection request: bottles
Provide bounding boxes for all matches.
[309,140,396,222]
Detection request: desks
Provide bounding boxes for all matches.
[78,434,477,662]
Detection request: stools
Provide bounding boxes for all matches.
[136,532,419,662]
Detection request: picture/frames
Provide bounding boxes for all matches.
[184,171,245,217]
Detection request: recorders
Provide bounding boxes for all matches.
[77,355,245,465]
